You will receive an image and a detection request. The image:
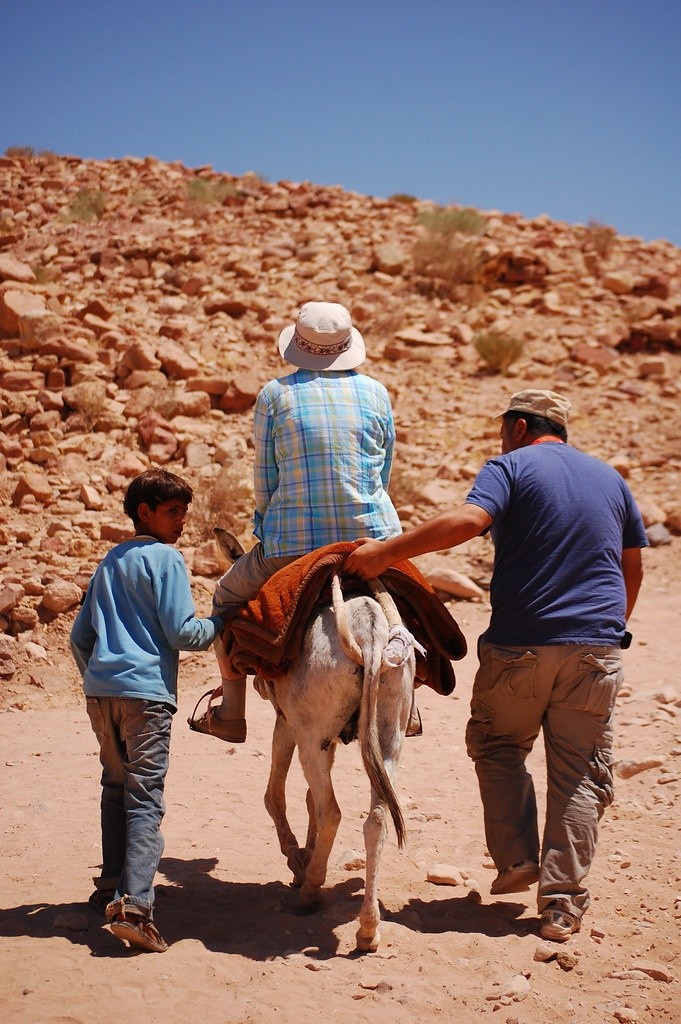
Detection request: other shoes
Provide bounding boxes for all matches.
[187,705,246,744]
[490,860,541,895]
[541,898,583,942]
[407,713,421,735]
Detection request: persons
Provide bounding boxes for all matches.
[68,469,238,951]
[343,391,649,941]
[186,301,420,744]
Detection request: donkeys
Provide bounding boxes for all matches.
[211,523,424,954]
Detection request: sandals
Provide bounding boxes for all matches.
[111,910,168,952]
[90,889,115,915]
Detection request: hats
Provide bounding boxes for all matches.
[278,301,367,371]
[495,389,569,428]
